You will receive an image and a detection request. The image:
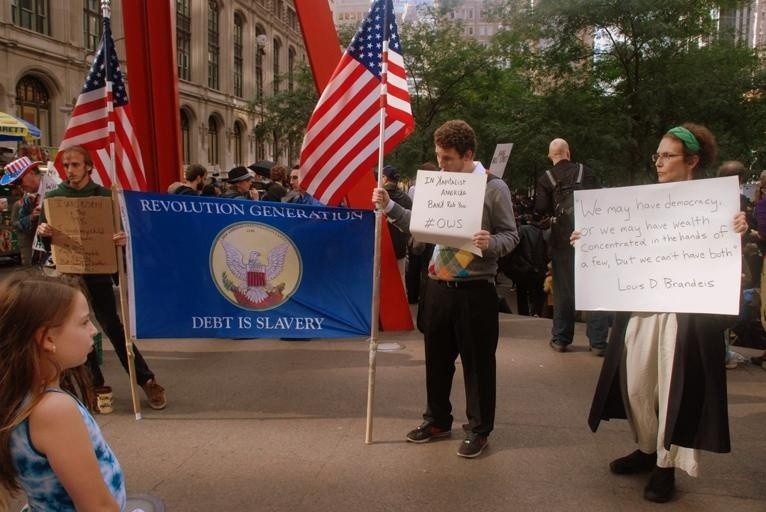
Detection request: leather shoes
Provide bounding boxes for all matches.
[141,374,172,410]
[546,338,567,353]
[588,342,609,356]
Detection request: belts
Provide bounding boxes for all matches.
[425,276,489,291]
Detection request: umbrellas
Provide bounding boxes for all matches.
[247,158,275,178]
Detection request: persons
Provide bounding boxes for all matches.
[261,164,288,203]
[751,170,766,369]
[0,269,127,510]
[172,164,207,198]
[531,139,609,355]
[372,120,519,458]
[717,159,752,368]
[407,162,434,198]
[219,167,258,200]
[513,223,547,317]
[512,190,549,228]
[374,166,411,305]
[1,154,58,231]
[282,168,320,206]
[200,171,223,197]
[569,123,756,503]
[36,145,168,409]
[543,260,553,317]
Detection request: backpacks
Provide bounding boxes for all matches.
[546,163,583,238]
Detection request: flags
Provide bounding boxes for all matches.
[116,188,383,336]
[297,1,416,209]
[55,1,147,195]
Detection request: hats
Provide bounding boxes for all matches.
[375,165,399,179]
[0,155,47,188]
[226,166,253,184]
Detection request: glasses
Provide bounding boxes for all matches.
[4,169,35,192]
[651,153,682,163]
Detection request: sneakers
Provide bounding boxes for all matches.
[608,448,658,476]
[405,418,452,444]
[642,465,678,504]
[457,423,489,458]
